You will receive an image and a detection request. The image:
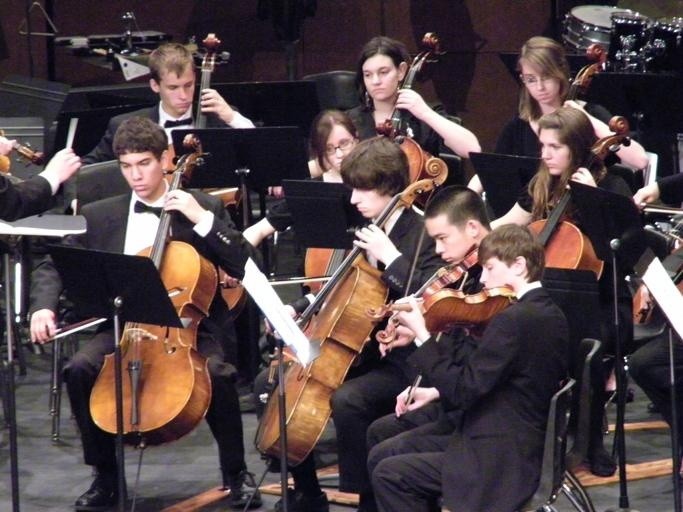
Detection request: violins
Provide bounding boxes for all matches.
[366,285,520,332]
[365,243,482,322]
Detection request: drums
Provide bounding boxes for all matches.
[606,12,651,63]
[562,5,637,56]
[650,15,683,72]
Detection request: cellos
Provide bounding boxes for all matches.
[151,32,248,322]
[254,159,451,467]
[304,31,446,302]
[89,133,218,446]
[527,114,633,281]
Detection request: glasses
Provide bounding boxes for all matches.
[323,139,353,155]
[521,73,552,87]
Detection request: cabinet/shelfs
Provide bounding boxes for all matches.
[633,247,683,347]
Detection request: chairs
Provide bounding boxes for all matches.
[439,154,461,185]
[303,71,360,110]
[449,116,462,126]
[565,338,602,512]
[523,377,586,512]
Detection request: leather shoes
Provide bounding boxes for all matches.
[74,467,128,509]
[272,489,331,511]
[229,467,262,507]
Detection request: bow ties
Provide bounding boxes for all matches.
[165,117,194,127]
[134,200,163,218]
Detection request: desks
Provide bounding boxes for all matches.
[586,72,683,177]
[500,53,593,88]
